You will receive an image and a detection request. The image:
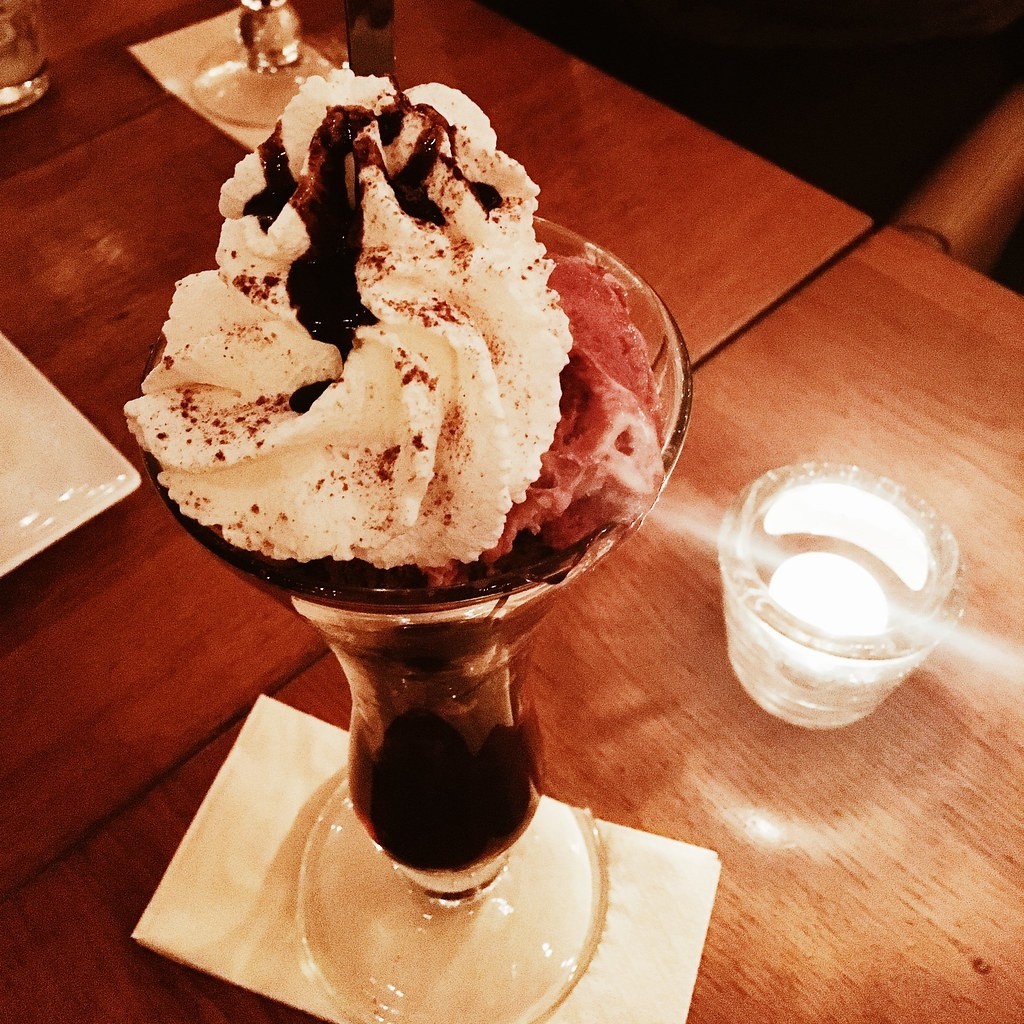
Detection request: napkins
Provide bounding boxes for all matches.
[127,8,335,153]
[135,690,721,1024]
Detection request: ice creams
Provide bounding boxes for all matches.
[119,69,671,603]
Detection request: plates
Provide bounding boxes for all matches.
[0,331,143,578]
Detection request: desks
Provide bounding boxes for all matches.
[0,0,1024,1024]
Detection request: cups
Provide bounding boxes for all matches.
[0,0,56,115]
[716,459,970,732]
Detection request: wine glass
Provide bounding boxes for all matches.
[189,0,338,131]
[142,214,696,1024]
